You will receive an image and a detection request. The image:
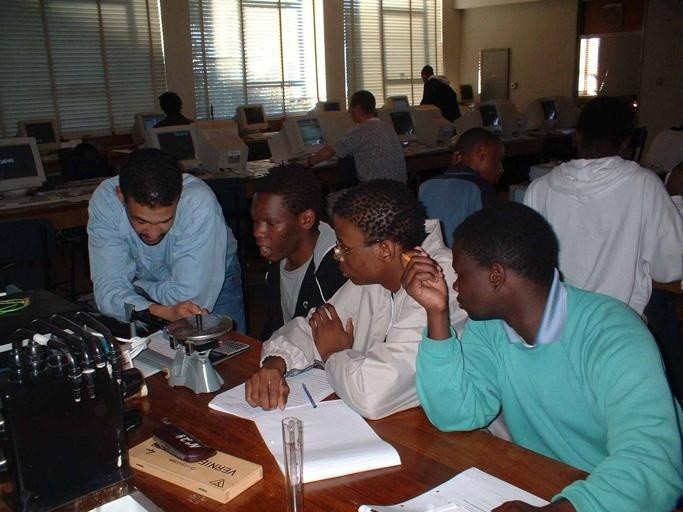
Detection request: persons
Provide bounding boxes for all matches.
[149,91,195,127]
[419,64,462,122]
[247,162,348,340]
[522,94,681,326]
[242,177,470,423]
[396,198,681,512]
[413,127,510,249]
[304,89,408,187]
[83,145,249,337]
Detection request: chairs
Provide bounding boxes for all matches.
[201,175,254,333]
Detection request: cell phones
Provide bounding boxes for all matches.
[209,340,250,366]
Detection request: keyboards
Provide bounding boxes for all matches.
[0,196,67,210]
[312,157,338,168]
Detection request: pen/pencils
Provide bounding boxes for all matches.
[402,253,411,262]
[302,383,316,408]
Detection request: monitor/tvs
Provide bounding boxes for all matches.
[266,113,326,160]
[0,136,47,200]
[140,125,203,172]
[16,119,60,161]
[131,112,167,151]
[233,104,268,137]
[194,119,249,173]
[313,83,563,154]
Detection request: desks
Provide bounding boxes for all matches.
[244,121,575,198]
[0,170,104,234]
[1,283,597,511]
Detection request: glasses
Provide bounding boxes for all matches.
[335,237,388,257]
